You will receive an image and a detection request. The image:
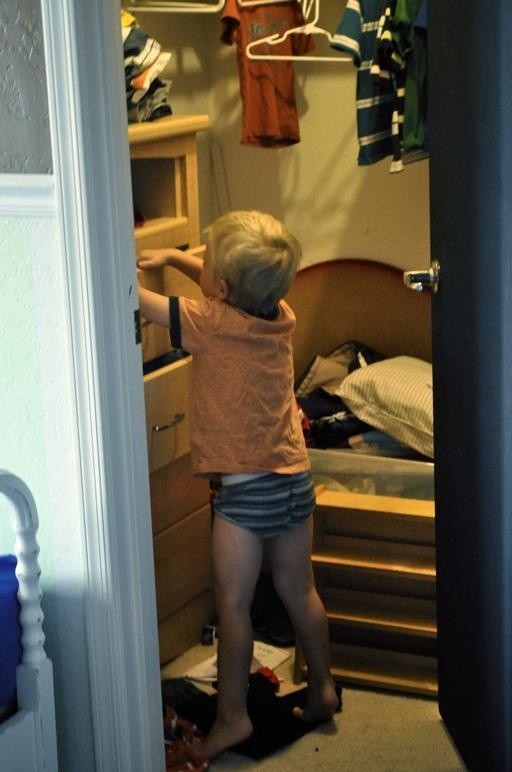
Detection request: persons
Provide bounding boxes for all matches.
[138,208,339,760]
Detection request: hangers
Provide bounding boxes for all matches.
[124,0,226,14]
[246,0,353,63]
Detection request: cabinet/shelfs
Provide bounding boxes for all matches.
[295,484,437,698]
[128,111,218,668]
